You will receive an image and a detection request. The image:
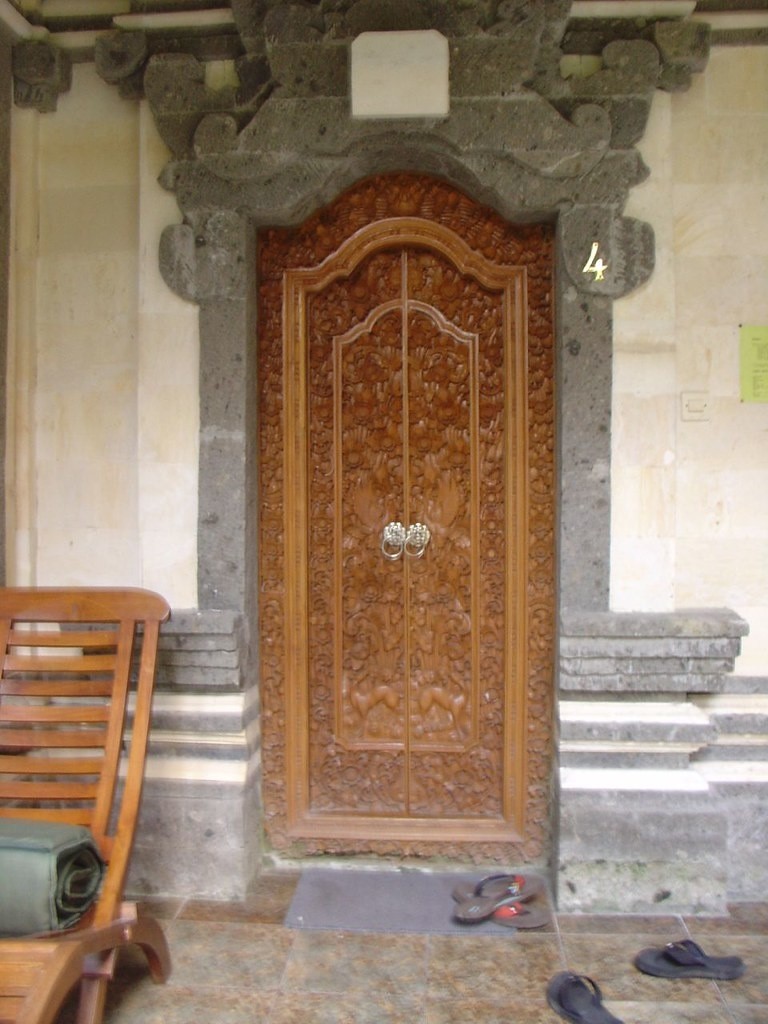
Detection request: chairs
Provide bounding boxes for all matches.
[0,586,172,1024]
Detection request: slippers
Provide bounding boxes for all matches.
[546,971,624,1024]
[454,872,542,922]
[633,939,744,979]
[452,884,551,928]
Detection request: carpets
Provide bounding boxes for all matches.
[283,868,519,936]
